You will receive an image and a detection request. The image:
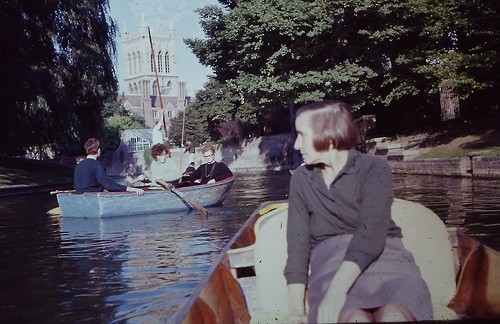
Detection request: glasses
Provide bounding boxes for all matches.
[203,152,214,157]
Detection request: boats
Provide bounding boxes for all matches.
[48,173,236,218]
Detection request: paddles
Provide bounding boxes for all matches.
[179,96,191,174]
[156,179,209,218]
[147,26,170,147]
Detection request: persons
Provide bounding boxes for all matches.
[283,100,434,324]
[185,144,233,187]
[149,144,182,186]
[74,138,145,196]
[186,162,196,173]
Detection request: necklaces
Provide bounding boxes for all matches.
[205,164,214,178]
